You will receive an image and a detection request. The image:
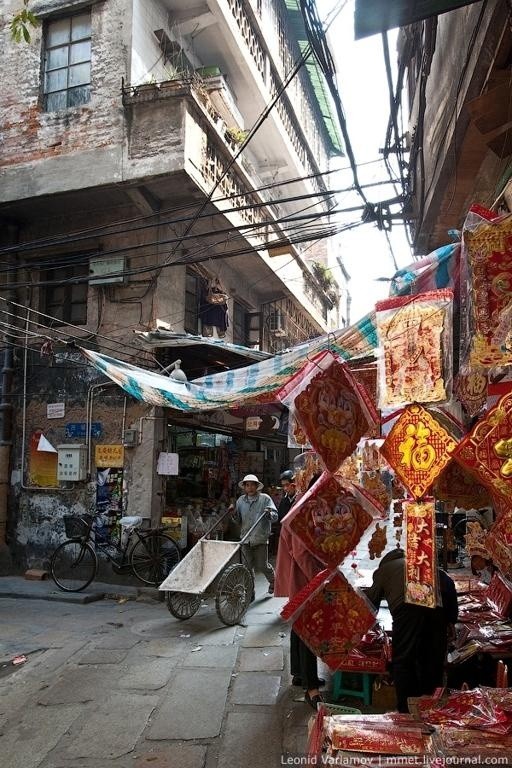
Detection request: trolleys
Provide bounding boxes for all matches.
[158,509,267,626]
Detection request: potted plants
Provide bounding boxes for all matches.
[132,63,188,96]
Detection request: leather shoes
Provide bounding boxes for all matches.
[292,676,325,686]
[305,690,325,712]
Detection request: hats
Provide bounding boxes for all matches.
[238,474,265,491]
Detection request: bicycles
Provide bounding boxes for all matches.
[51,508,181,592]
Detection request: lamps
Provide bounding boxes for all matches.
[159,355,187,382]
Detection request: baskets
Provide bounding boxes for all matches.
[317,702,361,718]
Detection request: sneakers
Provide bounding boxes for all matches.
[245,582,275,603]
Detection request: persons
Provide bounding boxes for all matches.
[273,471,328,711]
[278,468,298,536]
[226,475,280,604]
[366,548,460,714]
